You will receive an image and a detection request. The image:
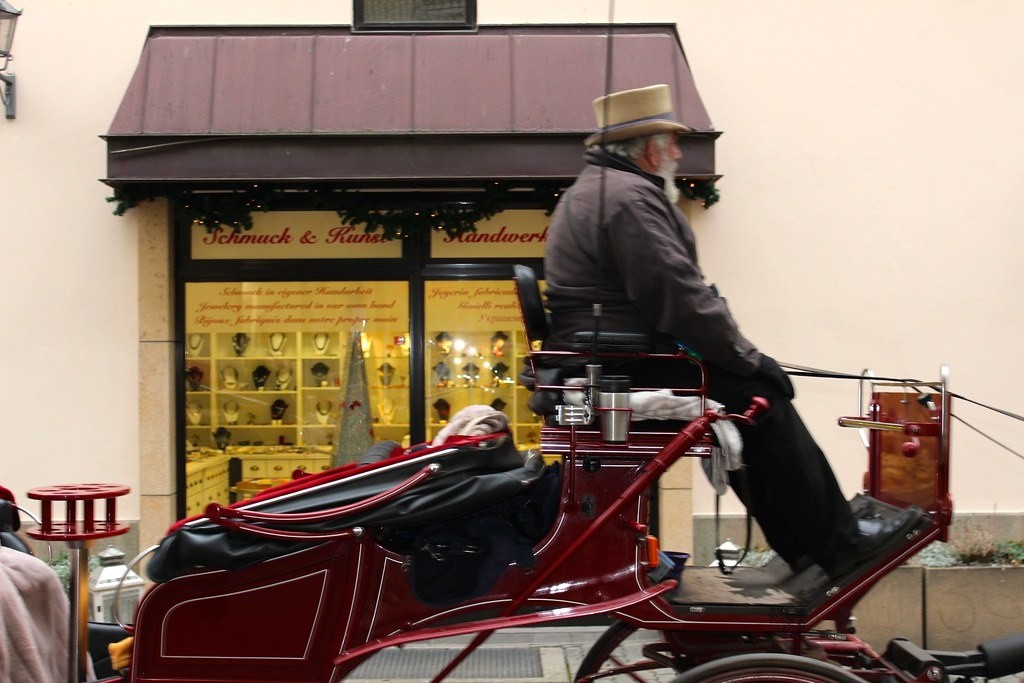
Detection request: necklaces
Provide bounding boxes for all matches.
[270,333,286,350]
[434,401,450,414]
[221,368,238,381]
[232,334,248,351]
[189,335,202,349]
[272,406,284,418]
[314,333,328,350]
[254,376,267,383]
[381,402,395,415]
[188,375,200,382]
[317,402,332,415]
[376,365,395,376]
[186,405,202,416]
[223,403,239,414]
[220,434,228,448]
[276,369,293,383]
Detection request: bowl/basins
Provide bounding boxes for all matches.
[650,550,691,580]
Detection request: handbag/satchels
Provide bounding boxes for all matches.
[143,430,563,606]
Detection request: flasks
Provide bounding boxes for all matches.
[598,375,631,444]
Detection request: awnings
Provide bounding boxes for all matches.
[98,31,724,195]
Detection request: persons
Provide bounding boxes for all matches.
[545,84,923,577]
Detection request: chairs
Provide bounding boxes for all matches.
[514,263,729,457]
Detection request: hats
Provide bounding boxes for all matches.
[0,484,22,532]
[584,83,699,147]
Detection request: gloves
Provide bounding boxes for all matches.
[753,352,795,400]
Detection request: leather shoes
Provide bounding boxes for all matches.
[817,508,922,581]
[846,493,876,519]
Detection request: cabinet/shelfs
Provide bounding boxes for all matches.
[423,278,566,468]
[186,278,413,519]
